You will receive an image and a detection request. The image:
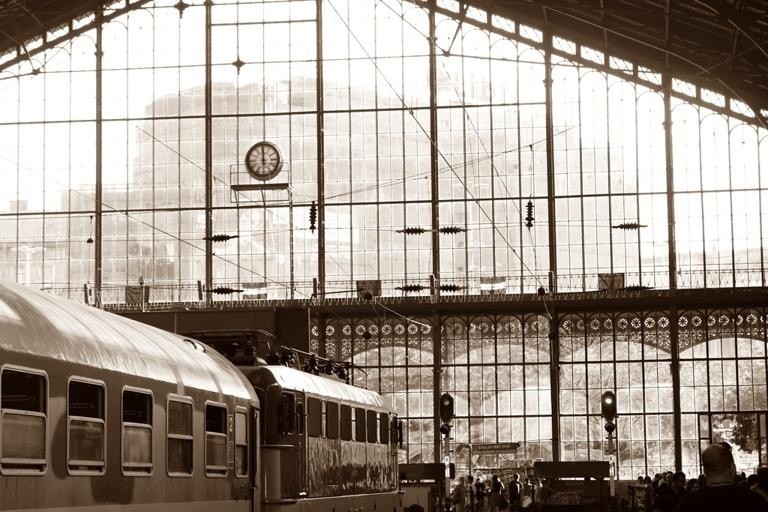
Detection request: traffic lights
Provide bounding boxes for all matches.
[599,390,616,434]
[438,392,454,433]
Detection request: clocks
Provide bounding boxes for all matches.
[244,141,283,180]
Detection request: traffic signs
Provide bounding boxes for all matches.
[472,443,518,454]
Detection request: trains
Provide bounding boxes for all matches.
[0,277,407,512]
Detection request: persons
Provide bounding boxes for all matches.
[636,465,768,512]
[677,443,767,511]
[450,473,543,512]
